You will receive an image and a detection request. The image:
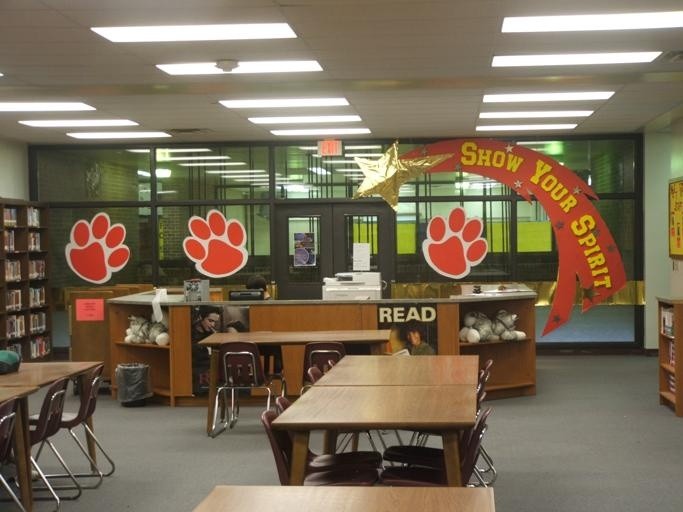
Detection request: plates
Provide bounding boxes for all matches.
[0,197,54,362]
[656,296,683,417]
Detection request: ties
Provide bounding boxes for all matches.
[229,291,264,301]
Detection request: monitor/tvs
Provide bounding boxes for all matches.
[29,287,47,333]
[668,375,676,394]
[660,307,674,337]
[29,260,46,280]
[8,343,22,359]
[5,261,21,283]
[27,208,40,251]
[29,336,50,360]
[4,290,24,338]
[4,208,17,250]
[667,341,674,366]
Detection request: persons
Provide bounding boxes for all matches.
[228,320,246,334]
[191,306,223,393]
[401,330,434,355]
[246,274,272,299]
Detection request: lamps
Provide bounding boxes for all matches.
[381,359,493,466]
[211,341,271,438]
[379,407,497,488]
[0,396,61,511]
[303,342,346,396]
[0,412,25,512]
[0,377,82,512]
[261,410,379,486]
[32,365,116,490]
[275,396,383,468]
[229,343,287,429]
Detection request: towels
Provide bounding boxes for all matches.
[116,363,149,406]
